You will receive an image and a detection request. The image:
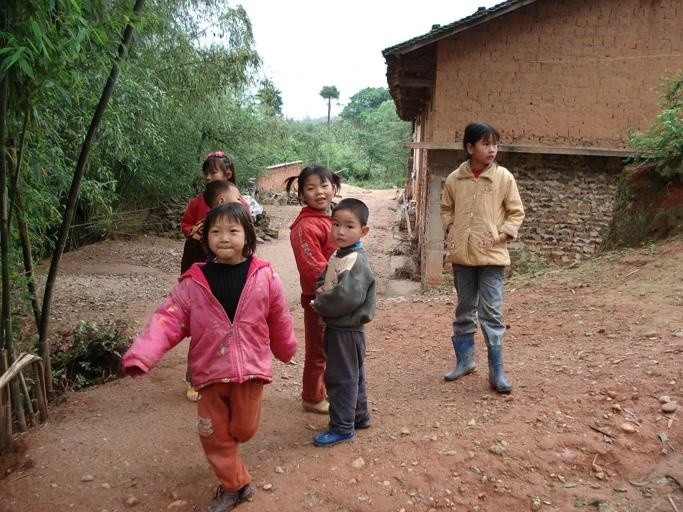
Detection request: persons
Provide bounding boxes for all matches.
[306,197,377,447]
[180,151,251,240]
[120,202,298,511]
[179,180,243,403]
[439,122,528,393]
[282,165,342,416]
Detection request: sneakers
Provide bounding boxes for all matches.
[209,482,252,511]
[354,419,370,430]
[312,428,355,448]
[302,400,329,413]
[187,385,198,401]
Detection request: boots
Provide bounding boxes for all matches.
[487,345,512,392]
[443,333,477,381]
